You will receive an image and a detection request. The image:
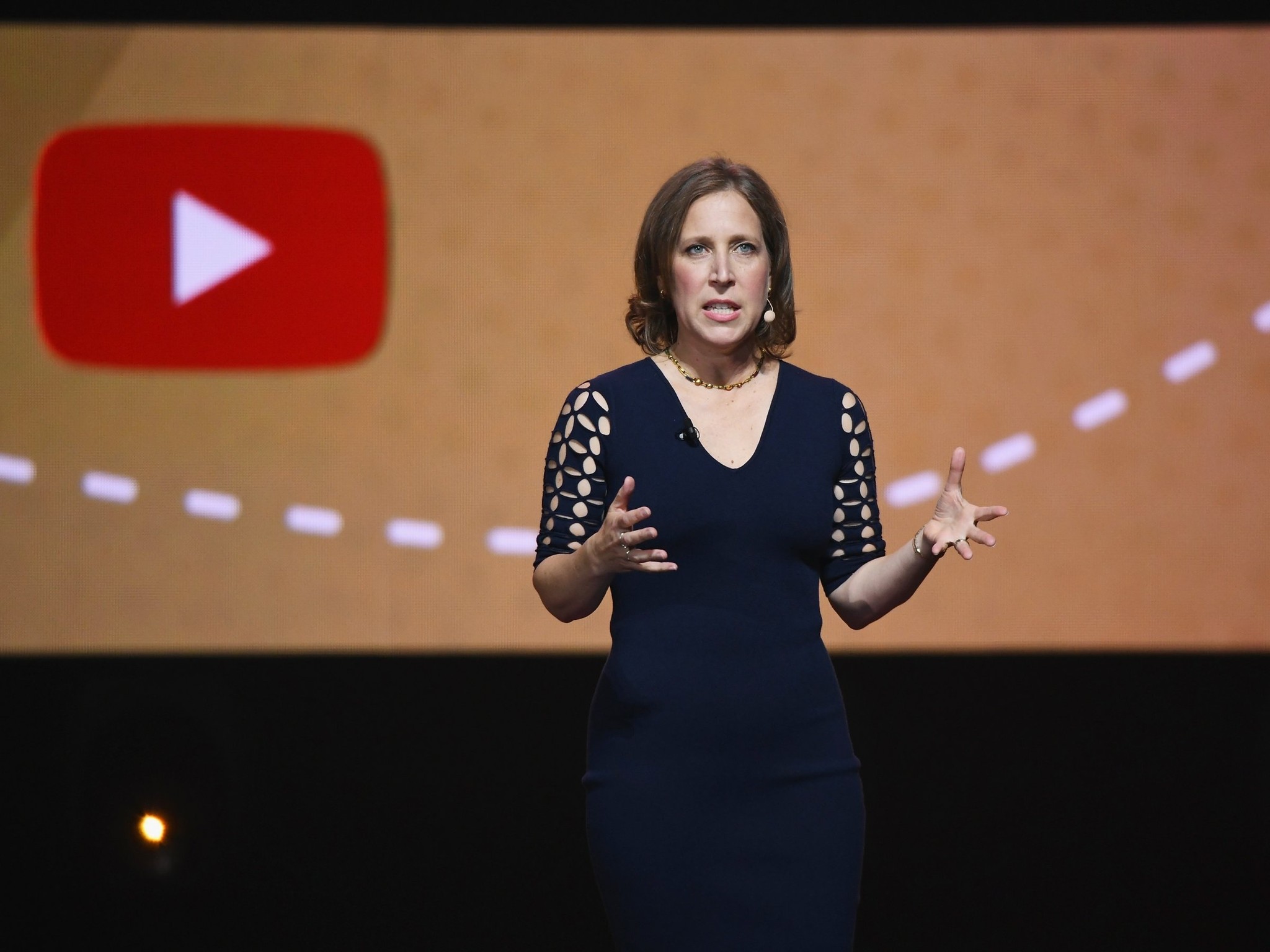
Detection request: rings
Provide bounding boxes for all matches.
[954,539,970,550]
[626,550,630,560]
[619,533,626,548]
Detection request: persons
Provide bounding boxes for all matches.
[531,156,1007,952]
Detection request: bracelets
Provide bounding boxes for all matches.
[912,529,946,558]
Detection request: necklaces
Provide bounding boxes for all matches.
[665,346,765,390]
